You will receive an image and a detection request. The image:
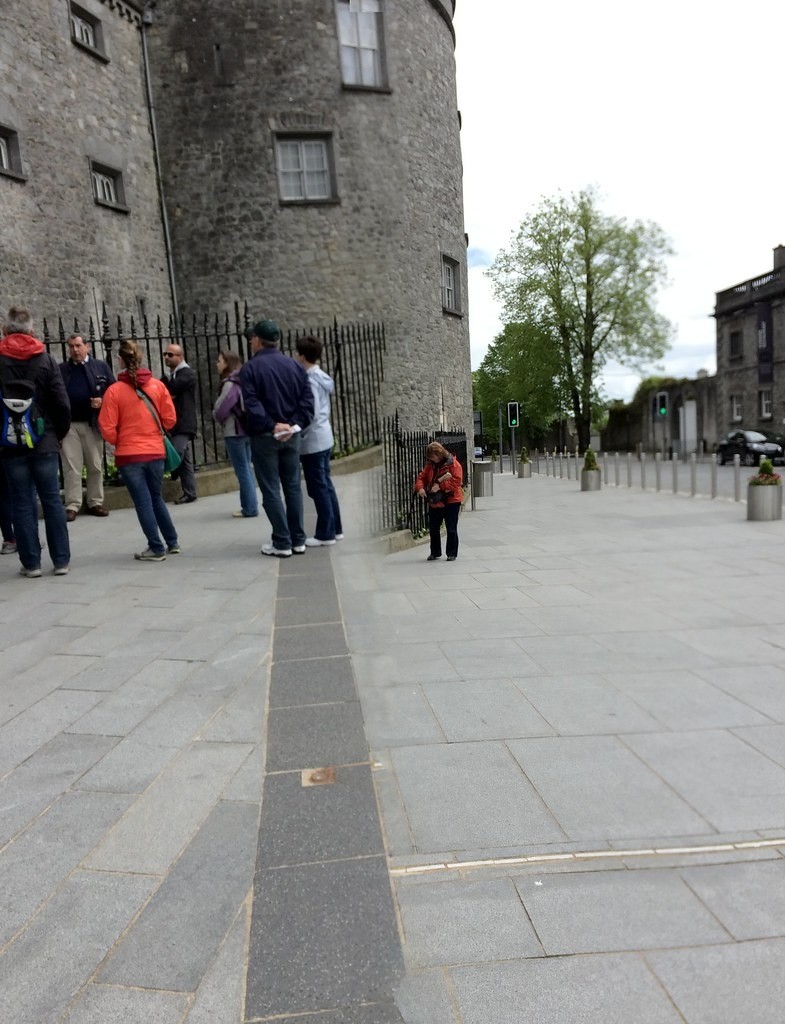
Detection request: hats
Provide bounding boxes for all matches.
[247,321,280,341]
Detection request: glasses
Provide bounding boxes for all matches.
[164,352,180,357]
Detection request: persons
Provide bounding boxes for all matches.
[288,334,346,549]
[96,343,182,565]
[0,307,72,580]
[55,334,116,522]
[414,439,466,567]
[159,344,198,505]
[211,350,261,521]
[238,318,317,560]
[667,445,678,460]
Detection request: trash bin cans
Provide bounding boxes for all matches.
[472,460,494,496]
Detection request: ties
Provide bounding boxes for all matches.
[169,373,174,381]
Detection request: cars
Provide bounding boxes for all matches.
[715,430,785,468]
[474,447,482,458]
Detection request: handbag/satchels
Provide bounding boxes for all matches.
[163,435,181,471]
[425,490,442,504]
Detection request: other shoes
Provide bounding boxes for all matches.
[175,493,195,504]
[1,541,17,553]
[54,564,69,574]
[87,505,108,515]
[67,510,75,520]
[447,556,457,561]
[304,534,344,546]
[40,542,45,548]
[169,545,180,553]
[428,555,436,560]
[20,565,41,577]
[261,544,306,557]
[232,511,244,517]
[134,547,166,560]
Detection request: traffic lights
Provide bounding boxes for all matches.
[656,392,668,417]
[508,402,519,427]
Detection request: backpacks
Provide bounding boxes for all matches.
[0,355,45,449]
